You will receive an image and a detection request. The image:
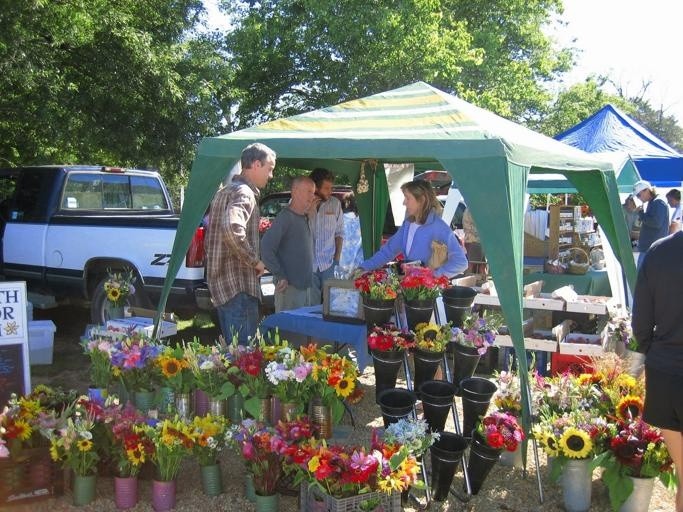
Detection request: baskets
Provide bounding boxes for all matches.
[543,246,605,275]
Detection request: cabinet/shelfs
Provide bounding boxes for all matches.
[523,202,602,258]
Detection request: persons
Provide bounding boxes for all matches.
[260,176,316,315]
[630,229,683,512]
[455,208,475,254]
[349,179,470,284]
[622,194,643,237]
[632,180,670,269]
[306,166,345,306]
[665,188,683,236]
[203,143,276,347]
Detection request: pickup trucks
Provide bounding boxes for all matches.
[0,164,275,328]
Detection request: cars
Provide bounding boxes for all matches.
[257,182,354,220]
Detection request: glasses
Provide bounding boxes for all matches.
[628,193,641,201]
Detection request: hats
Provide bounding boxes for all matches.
[631,180,652,198]
